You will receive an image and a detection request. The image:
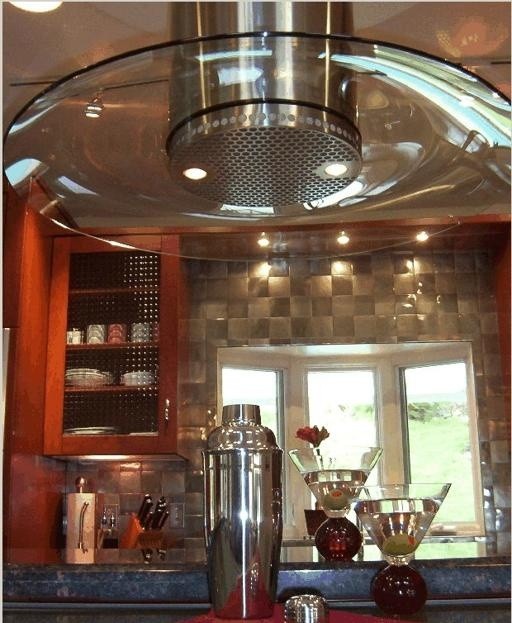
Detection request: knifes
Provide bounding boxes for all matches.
[137,493,170,530]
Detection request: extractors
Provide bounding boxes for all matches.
[4,0,512,262]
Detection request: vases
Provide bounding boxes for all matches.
[304,509,328,536]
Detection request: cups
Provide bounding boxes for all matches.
[66,322,160,344]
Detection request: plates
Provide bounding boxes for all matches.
[63,427,118,435]
[65,368,113,387]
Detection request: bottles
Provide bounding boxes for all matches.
[203,404,283,618]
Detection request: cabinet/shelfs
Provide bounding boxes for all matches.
[43,233,190,462]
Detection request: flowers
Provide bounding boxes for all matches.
[295,425,331,509]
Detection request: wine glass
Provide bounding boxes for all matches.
[287,448,451,614]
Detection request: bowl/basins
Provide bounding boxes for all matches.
[124,372,153,387]
